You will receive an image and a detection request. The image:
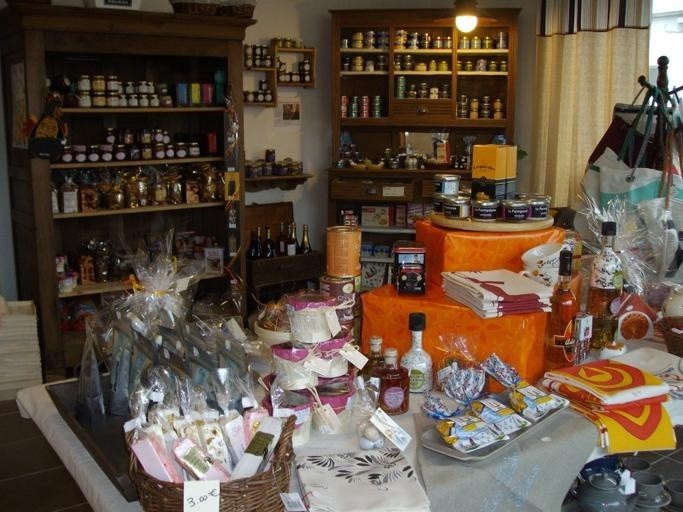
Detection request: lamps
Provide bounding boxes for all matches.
[430,0,499,35]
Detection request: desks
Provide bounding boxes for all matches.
[14,342,683,512]
[245,201,327,313]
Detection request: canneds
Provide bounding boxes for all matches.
[418,91,428,98]
[480,95,491,103]
[501,200,530,223]
[420,40,431,48]
[128,94,138,107]
[377,31,389,41]
[351,41,363,49]
[498,61,509,72]
[439,82,450,93]
[414,63,427,71]
[342,105,347,113]
[278,71,285,82]
[371,103,381,112]
[417,82,429,92]
[77,74,92,91]
[457,105,469,118]
[495,40,507,48]
[488,60,499,71]
[104,127,118,144]
[291,72,297,83]
[469,98,479,109]
[117,81,125,94]
[406,91,417,98]
[351,32,364,41]
[92,90,106,107]
[401,63,414,71]
[260,56,264,67]
[134,80,139,93]
[349,112,358,118]
[471,201,501,221]
[351,56,364,65]
[401,54,414,63]
[400,62,400,63]
[396,76,407,87]
[427,59,436,72]
[432,36,444,49]
[60,147,73,163]
[254,56,261,67]
[298,61,303,71]
[303,59,311,71]
[359,95,369,105]
[344,55,351,63]
[285,72,291,82]
[375,63,387,70]
[87,144,99,162]
[493,109,505,120]
[351,63,363,71]
[147,80,155,94]
[481,102,492,110]
[91,75,106,92]
[105,75,118,92]
[260,44,268,56]
[377,40,389,49]
[441,92,451,98]
[420,32,432,41]
[124,80,135,93]
[150,94,160,108]
[73,145,88,164]
[496,30,508,40]
[277,37,283,47]
[376,55,387,64]
[406,83,417,92]
[264,55,272,67]
[372,111,382,117]
[245,56,253,69]
[459,35,470,47]
[394,39,407,49]
[296,72,301,83]
[343,63,350,71]
[394,55,403,61]
[342,112,348,118]
[394,60,401,71]
[471,36,481,48]
[468,108,478,120]
[264,149,275,162]
[463,61,473,70]
[434,173,461,195]
[371,96,382,103]
[140,128,151,143]
[364,36,376,44]
[244,43,253,56]
[258,91,265,102]
[530,200,550,221]
[154,129,164,143]
[101,145,113,161]
[476,58,488,70]
[396,87,407,99]
[141,146,152,159]
[350,96,359,104]
[139,94,150,107]
[118,94,128,107]
[429,93,439,99]
[359,111,368,118]
[325,225,363,278]
[155,142,166,159]
[430,86,439,94]
[261,79,268,91]
[283,37,291,47]
[247,91,255,102]
[480,109,492,118]
[483,35,494,48]
[342,95,349,105]
[365,58,375,71]
[129,144,140,161]
[163,131,170,144]
[114,144,128,161]
[536,195,551,205]
[349,103,359,112]
[445,196,472,220]
[106,91,119,107]
[359,105,369,111]
[304,71,311,82]
[438,59,448,71]
[138,80,148,93]
[363,43,375,49]
[188,142,200,158]
[493,98,506,111]
[457,60,462,71]
[165,144,174,158]
[254,91,258,101]
[395,29,408,39]
[121,127,135,145]
[252,44,261,55]
[433,194,457,215]
[363,29,376,37]
[408,31,419,48]
[246,160,303,178]
[77,91,92,107]
[443,36,452,49]
[264,90,272,102]
[321,277,355,321]
[175,142,187,157]
[242,90,248,102]
[341,38,349,49]
[457,93,469,106]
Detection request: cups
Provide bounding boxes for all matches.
[189,84,201,106]
[203,84,214,105]
[665,479,683,507]
[176,82,188,107]
[520,242,571,269]
[519,268,558,287]
[616,457,651,473]
[207,133,218,156]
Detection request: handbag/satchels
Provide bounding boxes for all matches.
[638,197,683,285]
[583,116,659,173]
[574,147,631,245]
[663,130,683,177]
[599,168,683,211]
[612,104,674,138]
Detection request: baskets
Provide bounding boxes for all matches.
[124,414,297,512]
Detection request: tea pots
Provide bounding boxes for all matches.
[571,470,641,512]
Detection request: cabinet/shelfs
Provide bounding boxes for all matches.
[329,7,520,291]
[243,39,315,109]
[0,4,248,383]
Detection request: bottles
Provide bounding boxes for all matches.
[256,227,263,258]
[401,312,433,393]
[277,222,286,256]
[290,222,300,255]
[59,175,81,215]
[372,349,409,415]
[587,222,623,350]
[247,230,259,259]
[546,250,576,371]
[262,227,273,257]
[285,224,296,256]
[360,338,386,383]
[300,224,311,253]
[50,175,61,214]
